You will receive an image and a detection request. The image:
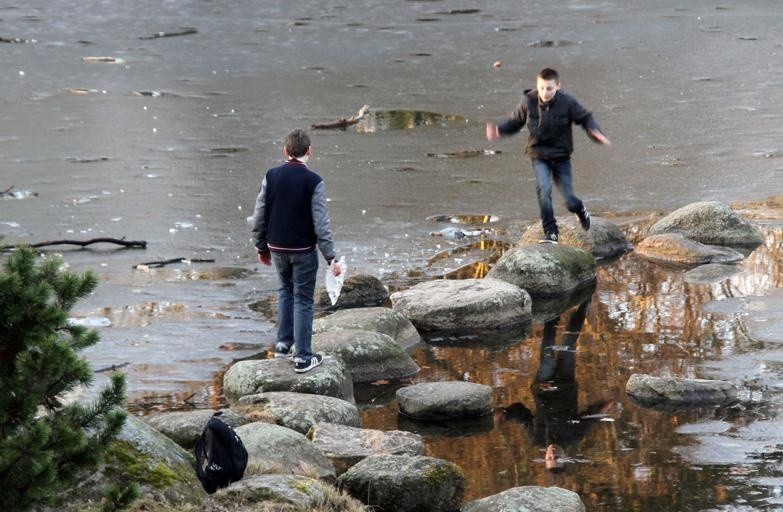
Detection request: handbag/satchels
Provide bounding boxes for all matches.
[194,418,248,494]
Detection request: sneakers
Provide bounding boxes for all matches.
[575,204,590,231]
[294,353,323,373]
[539,231,558,244]
[275,342,292,357]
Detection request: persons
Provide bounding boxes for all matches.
[490,291,613,477]
[484,66,612,242]
[248,128,343,377]
